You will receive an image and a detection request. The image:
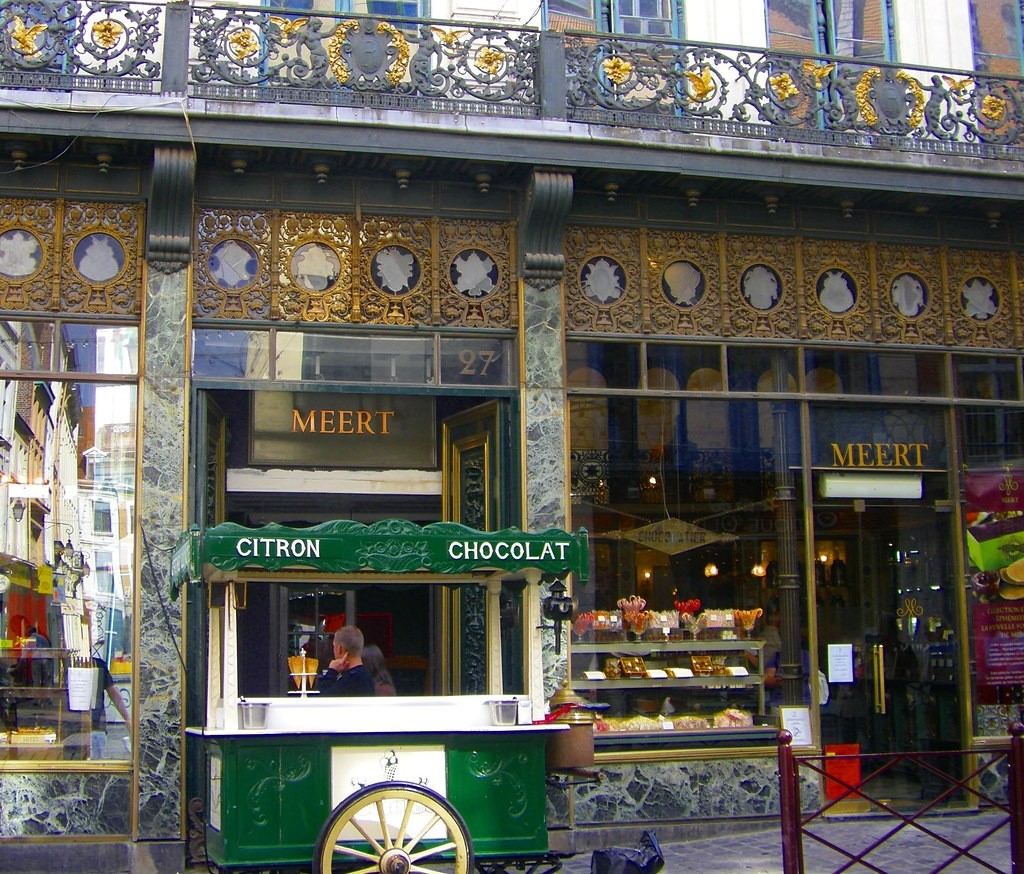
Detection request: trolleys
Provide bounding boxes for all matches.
[167,524,607,874]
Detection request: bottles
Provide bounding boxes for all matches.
[240,695,247,702]
[512,697,518,700]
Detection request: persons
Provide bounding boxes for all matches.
[300,636,336,670]
[748,597,810,728]
[64,647,132,734]
[318,625,374,697]
[869,616,919,781]
[361,644,397,697]
[16,626,53,708]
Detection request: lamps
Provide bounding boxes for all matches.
[43,520,75,560]
[74,550,91,578]
[12,497,25,522]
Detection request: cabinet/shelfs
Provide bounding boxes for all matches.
[0,645,92,760]
[567,638,769,727]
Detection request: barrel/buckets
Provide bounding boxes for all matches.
[488,700,519,726]
[238,702,272,729]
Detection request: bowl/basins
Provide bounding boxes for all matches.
[971,567,1000,595]
